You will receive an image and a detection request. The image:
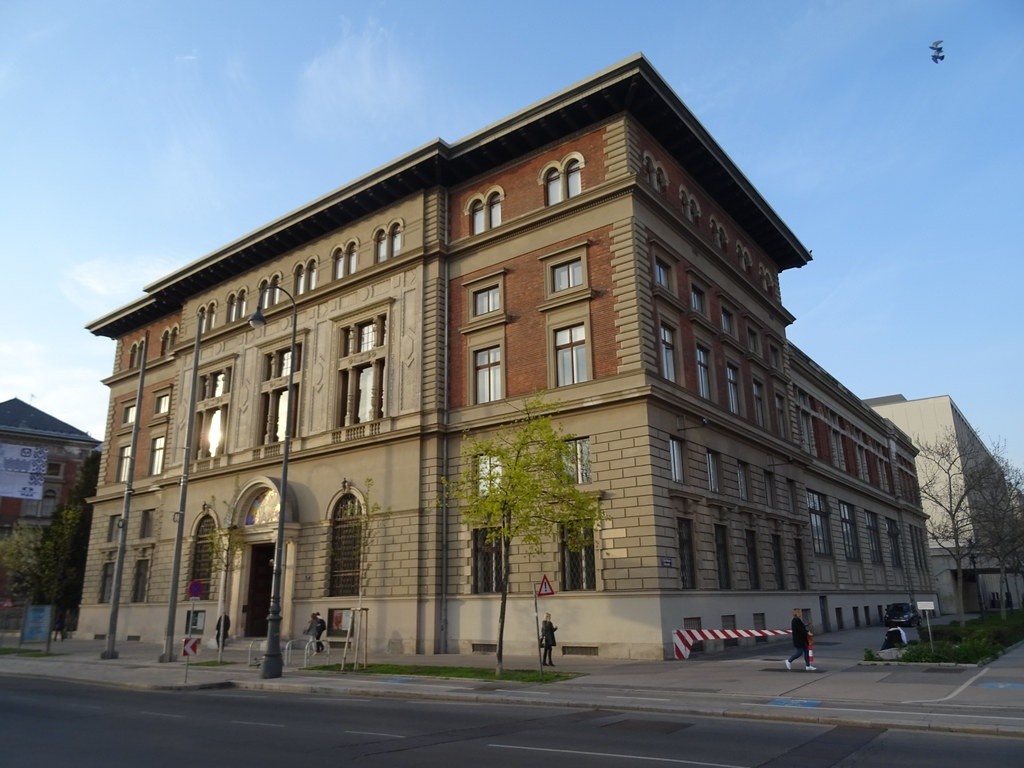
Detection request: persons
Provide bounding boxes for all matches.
[880,624,907,650]
[785,608,817,670]
[303,612,326,653]
[53,613,65,642]
[216,613,230,652]
[540,613,558,666]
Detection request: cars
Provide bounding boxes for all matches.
[884,602,922,628]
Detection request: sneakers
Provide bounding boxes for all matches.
[805,664,816,670]
[785,659,791,670]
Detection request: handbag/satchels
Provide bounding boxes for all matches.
[539,635,545,648]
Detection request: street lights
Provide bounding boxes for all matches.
[247,285,298,678]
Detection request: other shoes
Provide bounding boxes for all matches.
[549,662,554,666]
[543,662,549,666]
[312,652,318,656]
